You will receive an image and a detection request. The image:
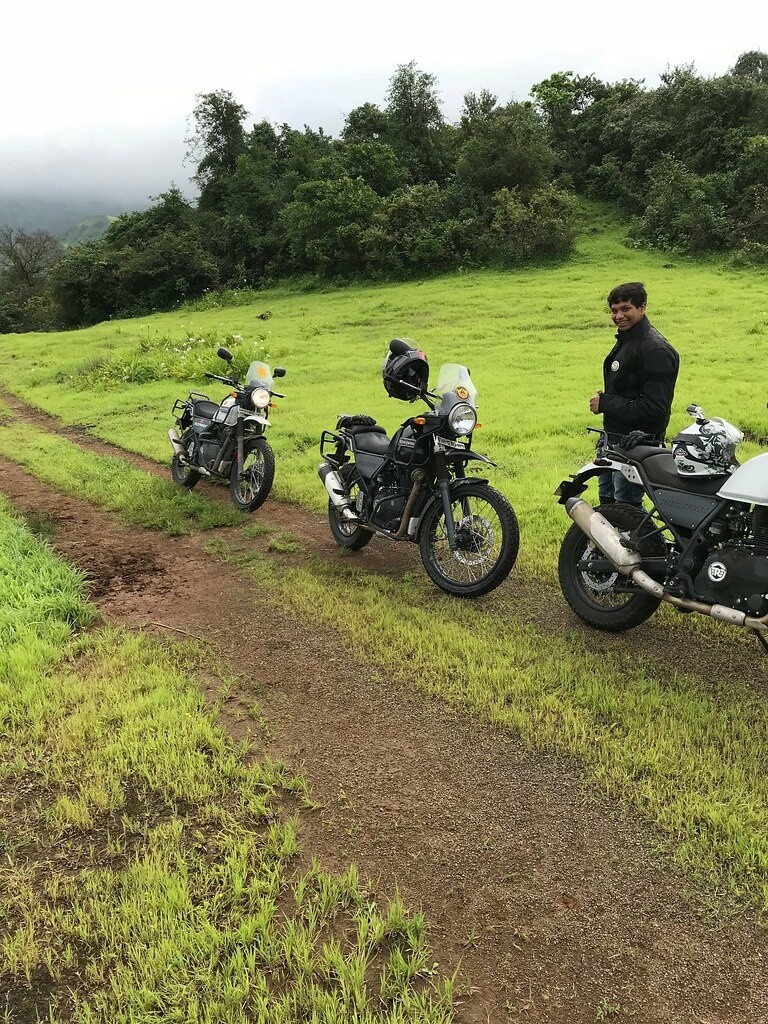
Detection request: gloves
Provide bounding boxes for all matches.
[336,414,376,430]
[619,430,659,450]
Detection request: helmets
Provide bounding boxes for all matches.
[382,350,429,403]
[671,404,744,478]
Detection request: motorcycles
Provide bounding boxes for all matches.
[554,428,768,652]
[166,347,288,511]
[318,339,521,598]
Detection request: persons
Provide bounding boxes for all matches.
[590,282,680,597]
